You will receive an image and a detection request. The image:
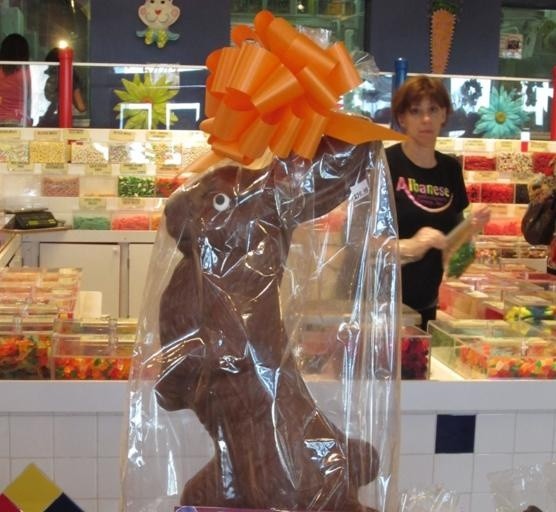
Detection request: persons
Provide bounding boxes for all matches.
[342,74,491,332]
[0,32,33,126]
[37,48,86,127]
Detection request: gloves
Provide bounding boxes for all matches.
[398,225,449,267]
[463,201,494,235]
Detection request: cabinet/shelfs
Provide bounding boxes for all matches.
[1,347,556,512]
[1,129,556,322]
